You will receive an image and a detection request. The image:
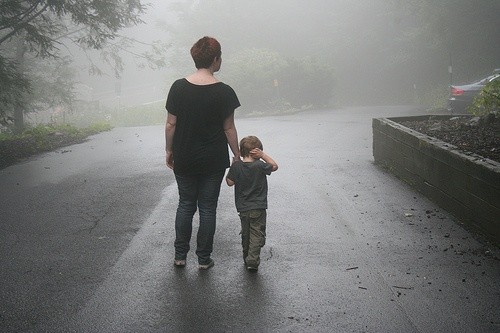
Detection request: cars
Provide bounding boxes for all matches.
[446,68,500,113]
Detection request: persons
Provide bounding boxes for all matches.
[226,135,278,270]
[166,37,241,269]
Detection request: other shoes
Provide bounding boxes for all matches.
[174,258,186,266]
[198,258,213,270]
[247,264,258,271]
